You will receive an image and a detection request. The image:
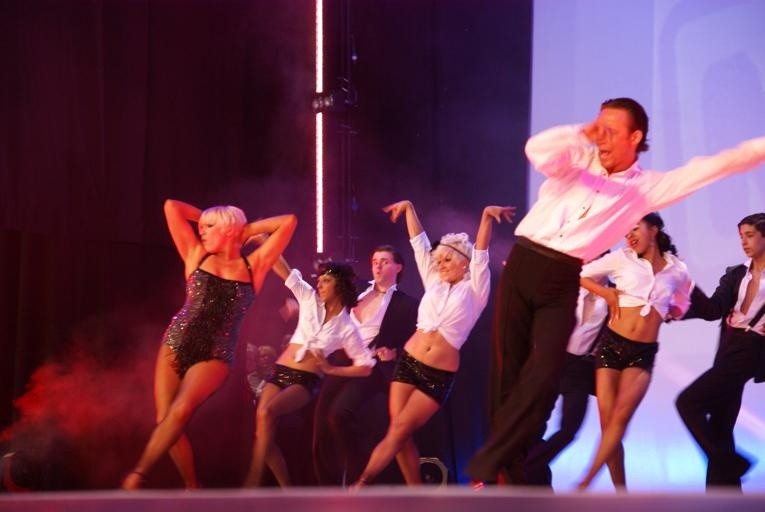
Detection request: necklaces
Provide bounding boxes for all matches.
[375,286,386,294]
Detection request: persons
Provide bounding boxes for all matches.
[462,95,765,492]
[575,210,696,496]
[674,212,765,497]
[238,233,377,491]
[310,244,420,488]
[119,198,299,492]
[530,248,616,487]
[346,199,517,493]
